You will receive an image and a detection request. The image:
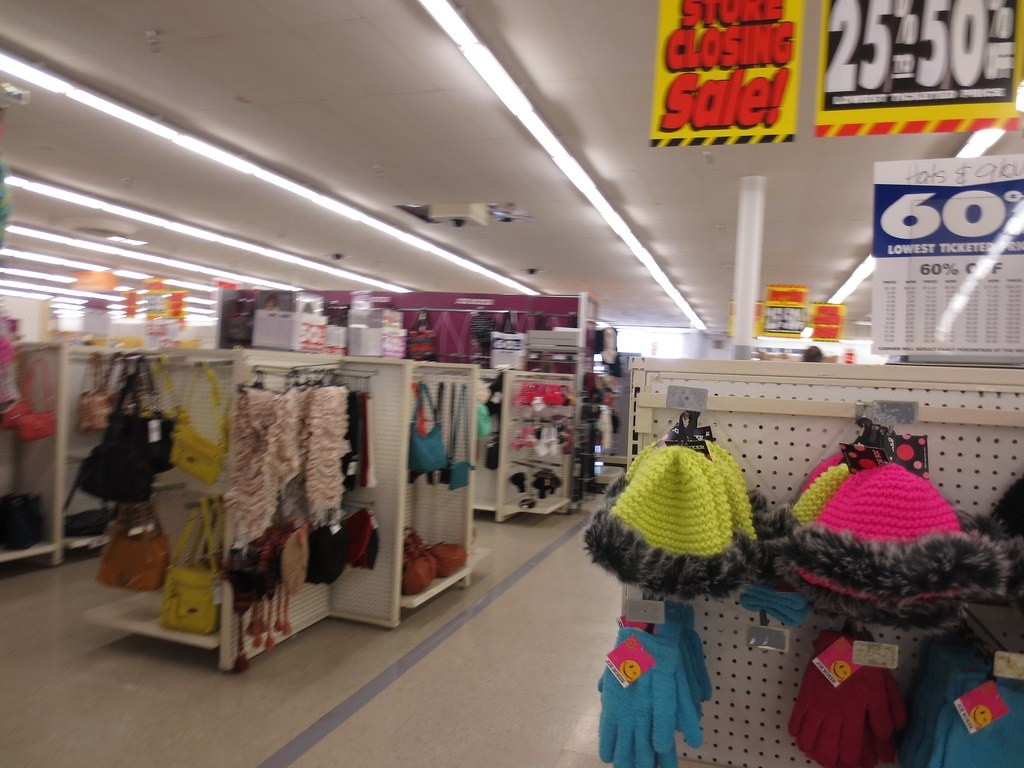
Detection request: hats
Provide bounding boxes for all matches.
[584,411,1009,625]
[226,505,381,673]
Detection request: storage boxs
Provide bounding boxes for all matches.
[251,310,408,361]
[487,326,577,371]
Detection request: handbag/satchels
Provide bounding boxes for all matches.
[61,350,226,635]
[1,351,56,441]
[408,379,473,490]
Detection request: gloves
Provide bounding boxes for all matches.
[597,598,712,768]
[788,626,1024,768]
